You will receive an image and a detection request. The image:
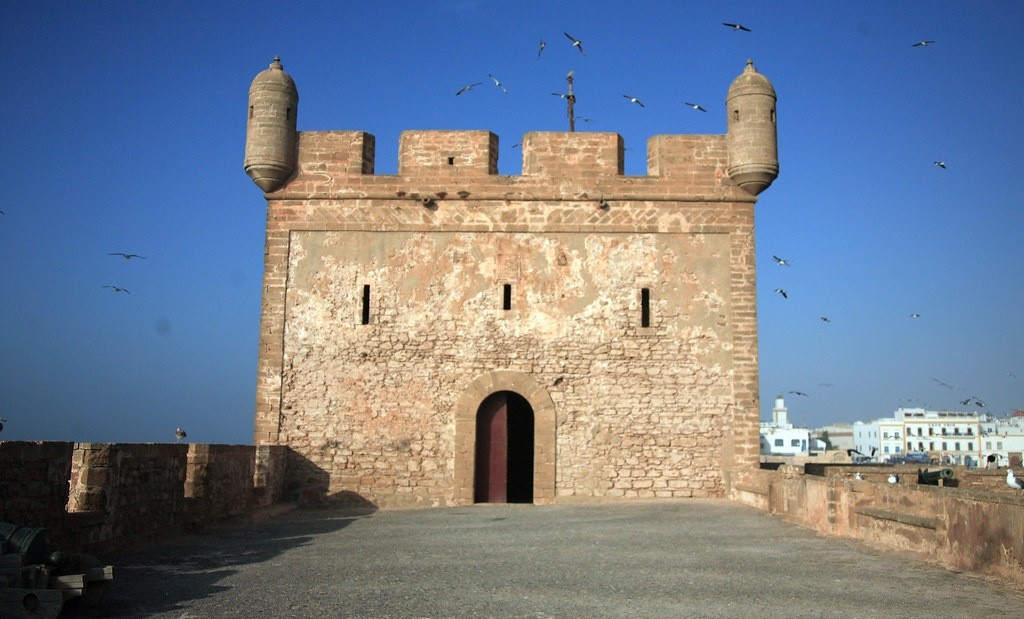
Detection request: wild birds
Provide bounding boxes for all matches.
[930,369,1022,407]
[787,390,806,397]
[886,473,900,485]
[774,288,788,299]
[685,102,708,113]
[455,81,482,97]
[1003,468,1024,490]
[107,252,148,261]
[909,314,921,318]
[488,73,509,94]
[623,94,646,108]
[575,114,596,123]
[550,90,576,103]
[912,41,937,47]
[175,425,186,444]
[934,160,947,169]
[563,31,585,57]
[536,39,547,60]
[772,255,791,267]
[820,316,833,325]
[723,21,751,32]
[102,285,133,296]
